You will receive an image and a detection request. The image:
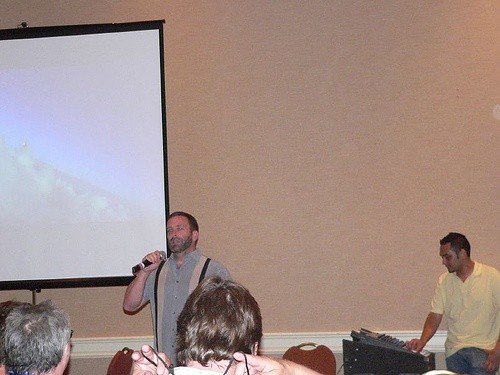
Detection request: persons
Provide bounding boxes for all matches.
[403,232,500,375]
[123,211,232,367]
[4,302,71,375]
[0,299,24,375]
[129,275,322,374]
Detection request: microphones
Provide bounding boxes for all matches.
[132,251,166,273]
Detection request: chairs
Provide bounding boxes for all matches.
[106,347,134,375]
[282,342,336,375]
